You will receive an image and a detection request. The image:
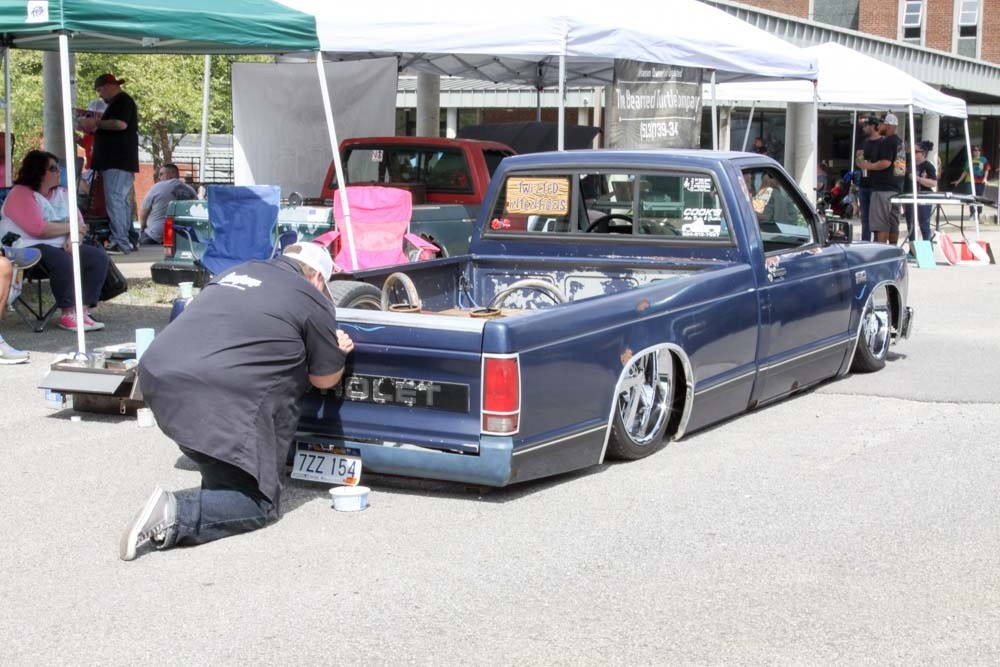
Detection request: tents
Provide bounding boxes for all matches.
[702,40,983,240]
[0,0,357,359]
[273,0,817,153]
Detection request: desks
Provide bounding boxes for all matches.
[890,198,984,249]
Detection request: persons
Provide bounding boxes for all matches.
[904,137,937,264]
[951,145,990,221]
[854,113,906,246]
[136,163,199,244]
[0,256,30,364]
[120,243,354,563]
[74,73,139,253]
[0,149,110,333]
[750,137,862,225]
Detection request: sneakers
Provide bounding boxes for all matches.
[82,313,104,330]
[120,487,169,561]
[58,312,89,331]
[0,339,30,364]
[0,246,41,269]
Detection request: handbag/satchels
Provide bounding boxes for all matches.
[82,239,127,301]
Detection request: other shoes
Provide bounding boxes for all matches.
[907,253,916,262]
[104,241,124,254]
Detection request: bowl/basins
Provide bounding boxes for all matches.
[329,486,370,512]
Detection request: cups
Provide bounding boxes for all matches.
[136,408,154,427]
[136,328,154,362]
[178,282,193,298]
[824,210,833,217]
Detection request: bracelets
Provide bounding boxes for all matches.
[919,177,922,184]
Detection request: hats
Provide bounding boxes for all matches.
[858,112,881,124]
[283,242,335,304]
[95,74,125,89]
[878,113,898,125]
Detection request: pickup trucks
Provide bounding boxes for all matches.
[151,137,518,288]
[171,150,914,487]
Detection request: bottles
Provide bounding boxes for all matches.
[863,160,871,178]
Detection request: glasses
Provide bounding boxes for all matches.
[973,149,980,151]
[159,172,166,175]
[915,150,924,153]
[44,165,59,172]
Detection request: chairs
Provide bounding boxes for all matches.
[11,259,58,333]
[172,184,298,292]
[310,185,440,272]
